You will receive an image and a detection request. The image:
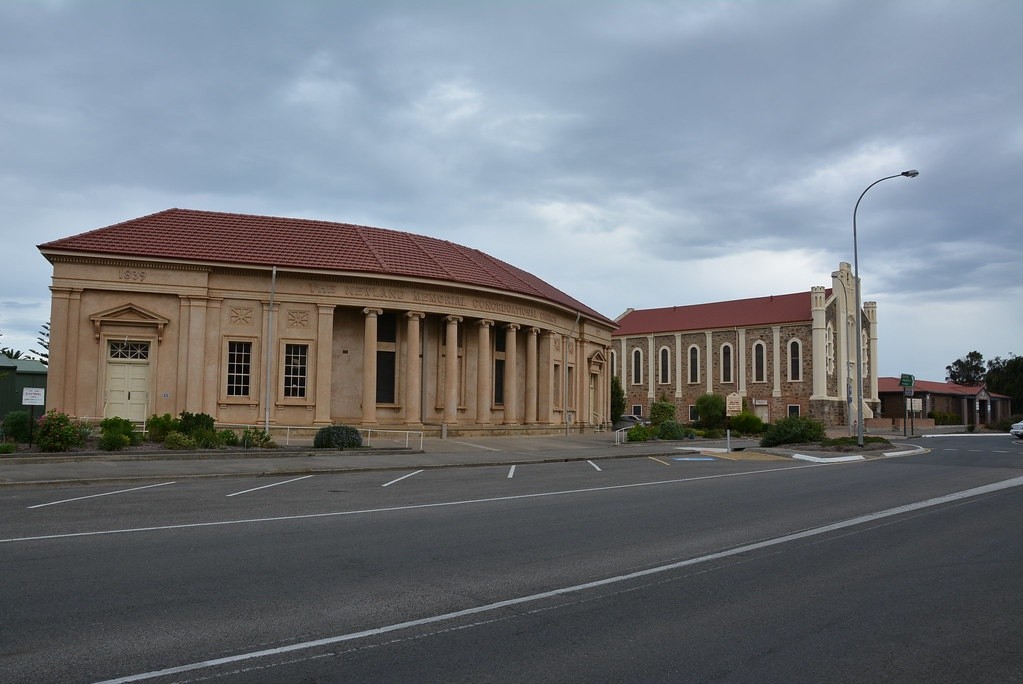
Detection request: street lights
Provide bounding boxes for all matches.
[853,170,919,447]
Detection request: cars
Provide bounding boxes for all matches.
[1010,421,1023,438]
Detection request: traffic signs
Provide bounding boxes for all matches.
[899,374,914,386]
